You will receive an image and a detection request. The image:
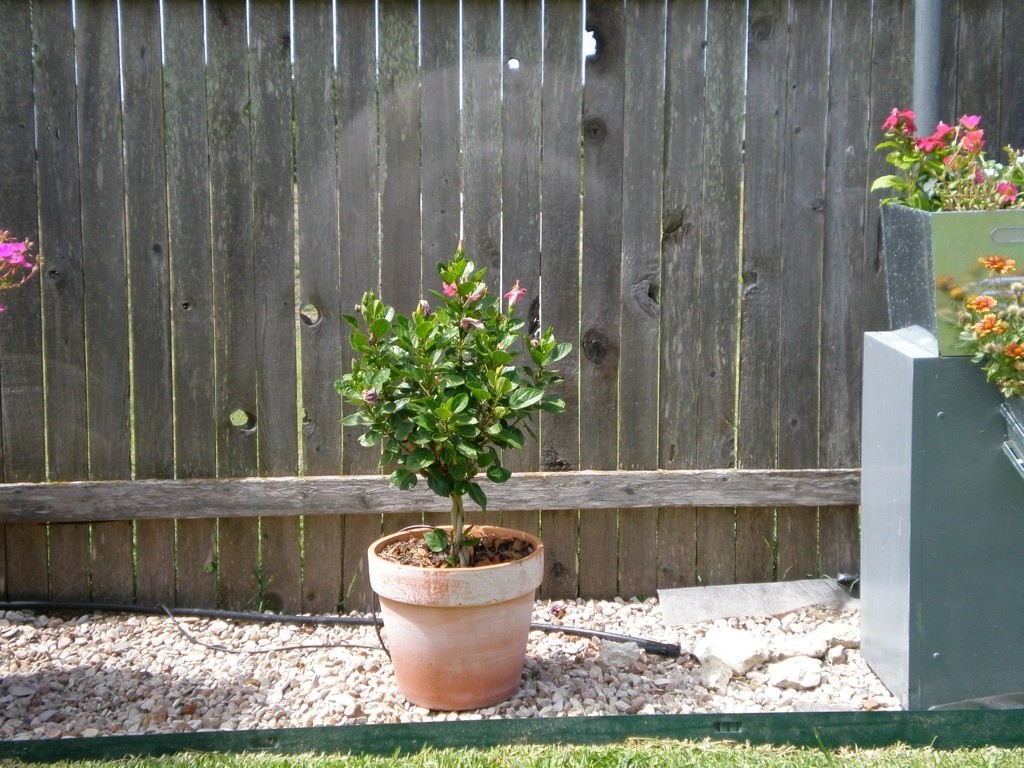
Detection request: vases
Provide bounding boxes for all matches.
[879,199,1024,357]
[1000,395,1024,478]
[368,524,544,714]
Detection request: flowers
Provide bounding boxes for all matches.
[0,228,44,314]
[950,255,1024,398]
[936,263,1024,356]
[869,107,1024,211]
[334,238,572,567]
